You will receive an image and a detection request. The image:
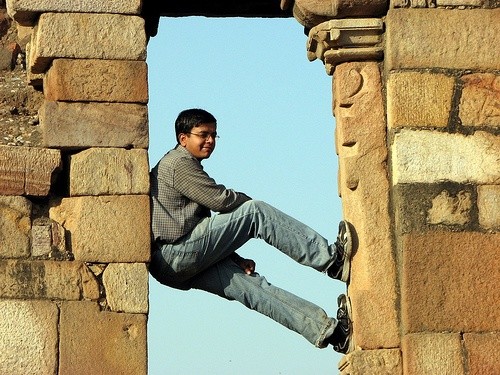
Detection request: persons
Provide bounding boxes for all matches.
[149,108,353,353]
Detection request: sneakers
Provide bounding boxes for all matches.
[328,220,352,281]
[333,293,352,355]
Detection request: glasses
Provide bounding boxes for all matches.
[187,132,216,138]
